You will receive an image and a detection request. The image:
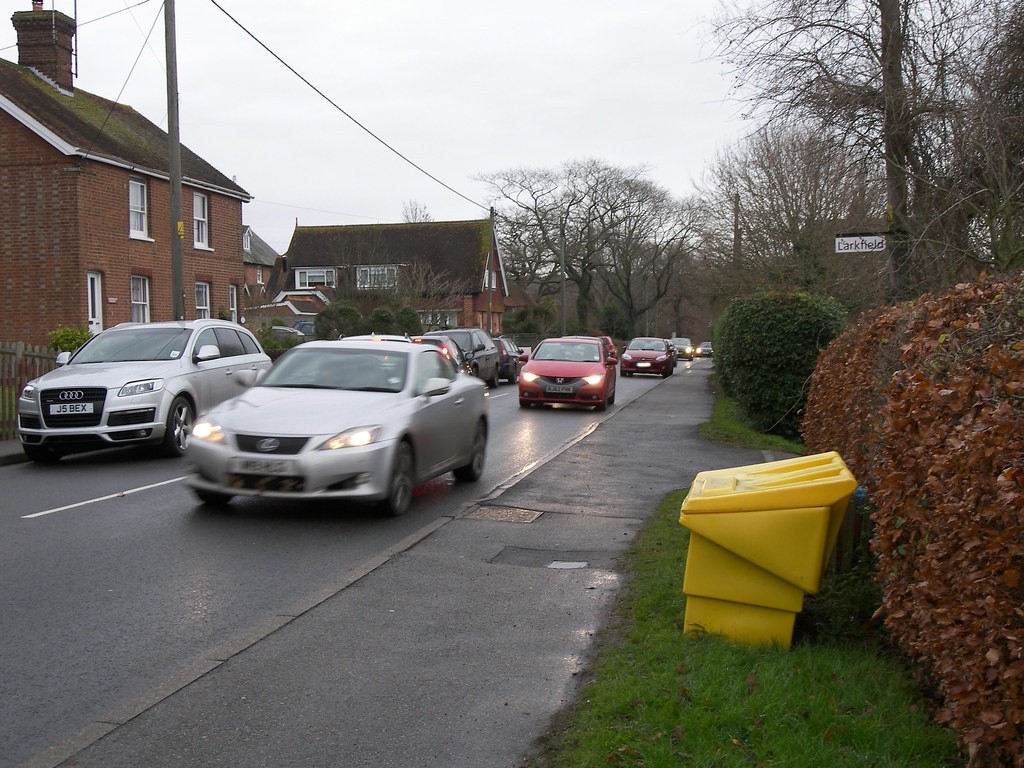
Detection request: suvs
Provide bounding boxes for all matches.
[670,336,695,361]
[16,318,273,464]
[291,319,317,337]
[336,326,527,388]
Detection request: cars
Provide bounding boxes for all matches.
[620,336,674,378]
[561,332,618,359]
[664,339,679,367]
[255,324,304,337]
[517,339,620,411]
[696,341,714,358]
[182,339,491,520]
